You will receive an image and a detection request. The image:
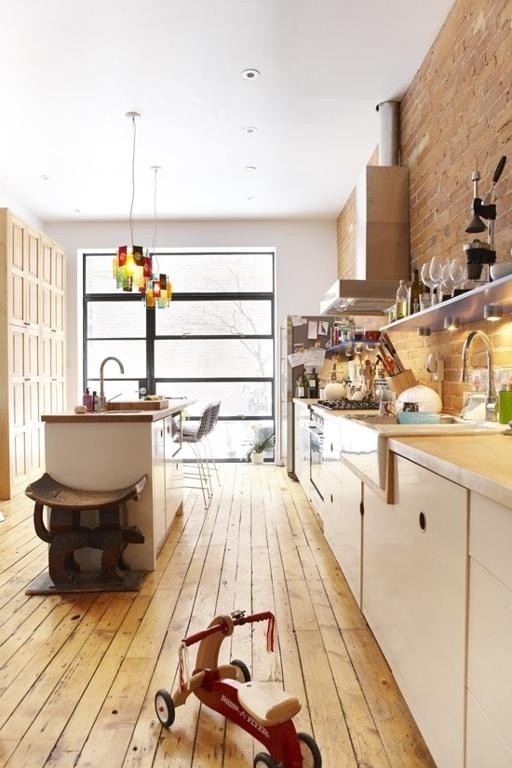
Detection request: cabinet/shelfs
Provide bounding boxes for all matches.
[309,402,341,551]
[0,331,38,431]
[292,398,312,503]
[338,414,384,658]
[0,207,41,331]
[41,399,196,573]
[0,432,40,501]
[383,434,512,768]
[38,332,67,427]
[41,231,66,337]
[379,270,512,337]
[38,427,46,473]
[325,340,381,359]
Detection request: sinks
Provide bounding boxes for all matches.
[356,414,462,426]
[102,409,143,415]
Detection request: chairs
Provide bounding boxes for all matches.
[173,403,213,511]
[178,400,222,496]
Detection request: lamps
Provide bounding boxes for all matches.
[141,165,173,309]
[112,111,153,295]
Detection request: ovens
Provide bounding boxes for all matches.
[310,412,324,514]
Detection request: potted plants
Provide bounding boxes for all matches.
[240,430,276,465]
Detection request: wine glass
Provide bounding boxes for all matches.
[449,257,467,297]
[429,254,451,304]
[442,265,457,298]
[420,262,439,307]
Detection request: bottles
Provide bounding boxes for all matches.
[396,279,408,318]
[92,391,97,411]
[308,369,318,398]
[406,269,422,313]
[299,370,308,398]
[83,387,92,411]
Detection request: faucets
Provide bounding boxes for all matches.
[99,356,124,403]
[459,331,500,423]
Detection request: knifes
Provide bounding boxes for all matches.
[382,333,404,370]
[376,333,405,375]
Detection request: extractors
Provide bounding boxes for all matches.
[319,165,411,317]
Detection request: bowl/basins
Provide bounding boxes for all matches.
[489,262,512,281]
[399,412,441,425]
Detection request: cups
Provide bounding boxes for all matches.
[498,390,512,424]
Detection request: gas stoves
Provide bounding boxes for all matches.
[317,399,380,411]
